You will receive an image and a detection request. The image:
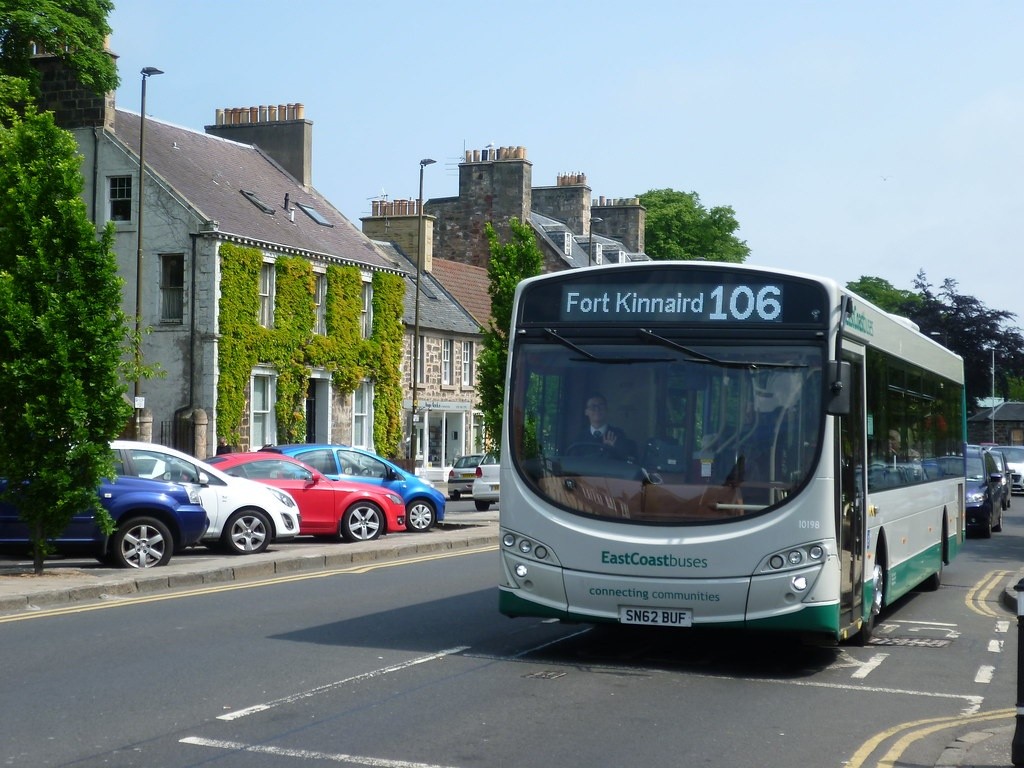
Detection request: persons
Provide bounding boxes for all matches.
[563,393,626,460]
[882,430,920,463]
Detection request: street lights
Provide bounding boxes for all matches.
[588,215,603,267]
[407,156,438,473]
[989,345,998,446]
[133,60,166,420]
[930,331,948,349]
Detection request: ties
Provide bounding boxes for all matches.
[594,431,603,443]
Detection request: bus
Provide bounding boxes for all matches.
[498,255,969,656]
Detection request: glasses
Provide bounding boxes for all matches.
[587,403,606,411]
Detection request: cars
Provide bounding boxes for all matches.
[258,443,448,533]
[988,445,1024,497]
[967,445,1004,538]
[447,453,485,500]
[986,450,1012,510]
[152,450,407,542]
[472,450,500,512]
[0,473,211,568]
[66,440,301,553]
